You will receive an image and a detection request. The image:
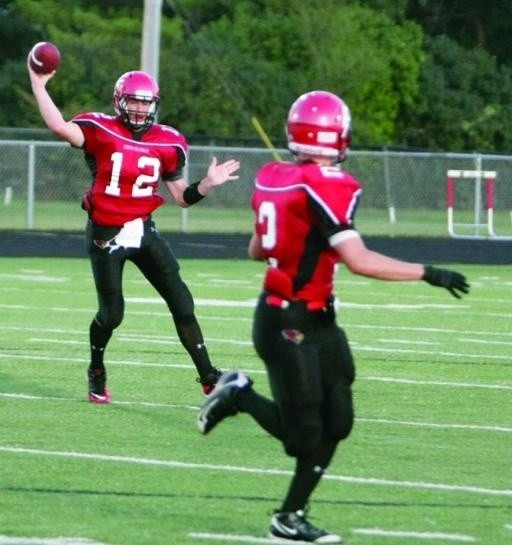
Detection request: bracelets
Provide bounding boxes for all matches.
[183,181,204,206]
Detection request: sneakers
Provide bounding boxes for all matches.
[264,508,342,544]
[87,361,111,405]
[197,370,253,435]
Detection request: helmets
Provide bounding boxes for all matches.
[284,91,353,162]
[112,69,160,136]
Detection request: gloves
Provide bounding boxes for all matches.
[422,266,471,300]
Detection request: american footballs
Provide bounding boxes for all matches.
[30,41,62,74]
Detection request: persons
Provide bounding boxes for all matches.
[197,89,472,544]
[25,42,243,408]
